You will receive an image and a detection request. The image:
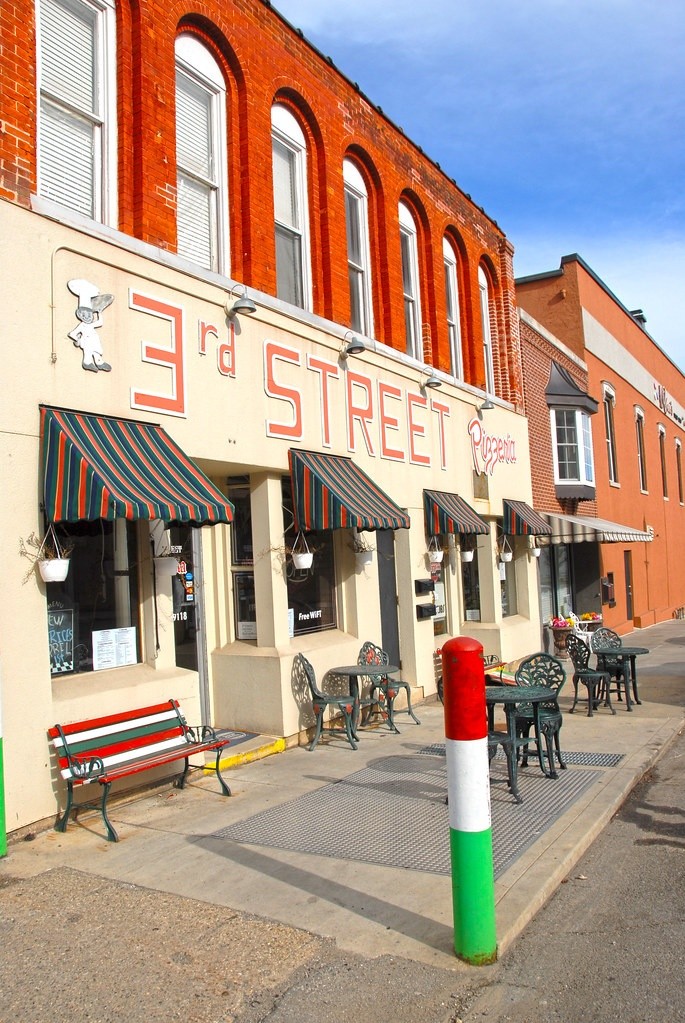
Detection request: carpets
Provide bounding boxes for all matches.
[202,729,261,752]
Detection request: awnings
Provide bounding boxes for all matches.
[537,512,652,545]
[287,449,411,533]
[503,499,554,535]
[38,406,236,530]
[423,489,491,535]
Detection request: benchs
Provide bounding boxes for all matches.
[432,647,507,696]
[47,697,232,843]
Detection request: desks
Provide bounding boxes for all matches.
[328,665,400,741]
[593,647,649,711]
[484,687,557,792]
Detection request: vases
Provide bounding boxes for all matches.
[549,627,574,658]
[37,558,71,583]
[426,551,444,562]
[292,552,314,569]
[500,552,512,562]
[153,556,181,576]
[529,548,541,557]
[585,620,604,634]
[460,551,474,562]
[355,551,372,565]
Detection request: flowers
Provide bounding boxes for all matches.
[550,618,574,628]
[579,611,600,621]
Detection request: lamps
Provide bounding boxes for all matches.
[224,284,257,317]
[340,331,365,360]
[475,392,495,412]
[419,367,442,389]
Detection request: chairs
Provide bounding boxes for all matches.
[358,641,421,734]
[564,634,616,717]
[436,675,523,804]
[592,627,635,707]
[515,651,567,768]
[559,614,587,652]
[570,612,596,653]
[299,653,360,750]
